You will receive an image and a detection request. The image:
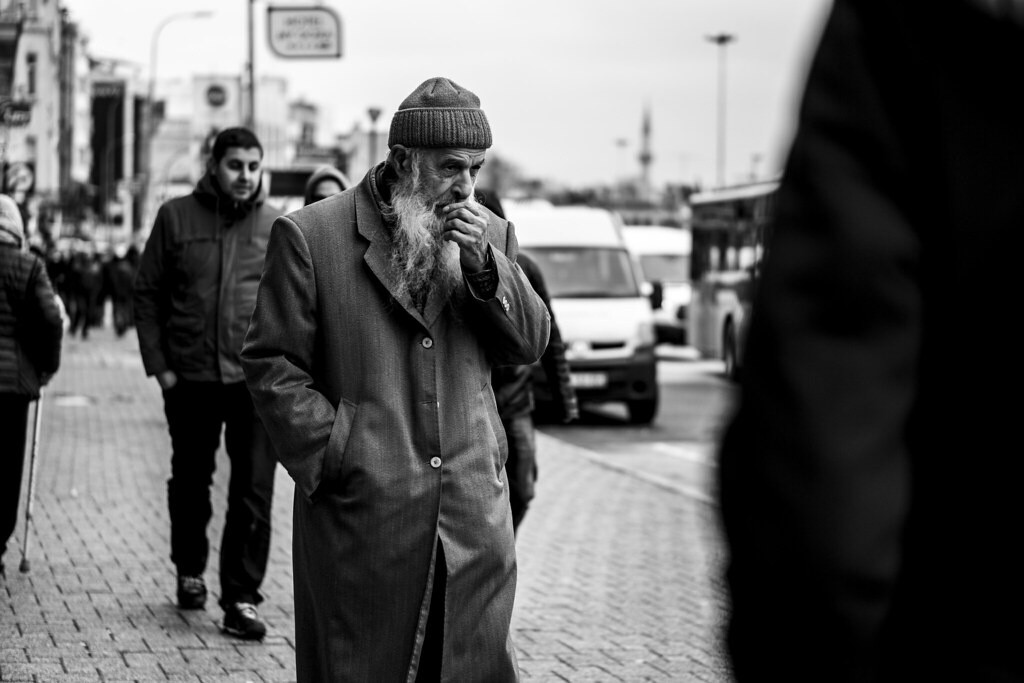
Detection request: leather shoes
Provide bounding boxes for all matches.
[224,602,266,640]
[178,574,207,609]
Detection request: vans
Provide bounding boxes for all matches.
[508,208,665,427]
[624,226,694,344]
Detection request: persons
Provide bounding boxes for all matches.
[135,126,284,640]
[305,165,350,210]
[0,193,64,573]
[44,240,139,340]
[717,0,1024,683]
[474,188,579,535]
[239,78,552,683]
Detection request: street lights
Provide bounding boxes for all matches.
[138,9,210,223]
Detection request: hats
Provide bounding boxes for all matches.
[388,77,492,149]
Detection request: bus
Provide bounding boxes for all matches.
[691,180,786,378]
[260,167,316,214]
